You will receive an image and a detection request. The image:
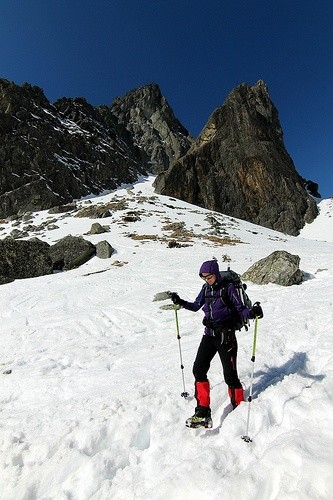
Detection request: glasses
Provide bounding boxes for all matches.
[201,273,213,280]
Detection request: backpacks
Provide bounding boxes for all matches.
[220,270,251,331]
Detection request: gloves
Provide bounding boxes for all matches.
[249,306,263,319]
[171,293,181,305]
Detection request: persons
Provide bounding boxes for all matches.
[170,261,264,429]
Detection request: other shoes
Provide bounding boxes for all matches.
[185,415,214,429]
